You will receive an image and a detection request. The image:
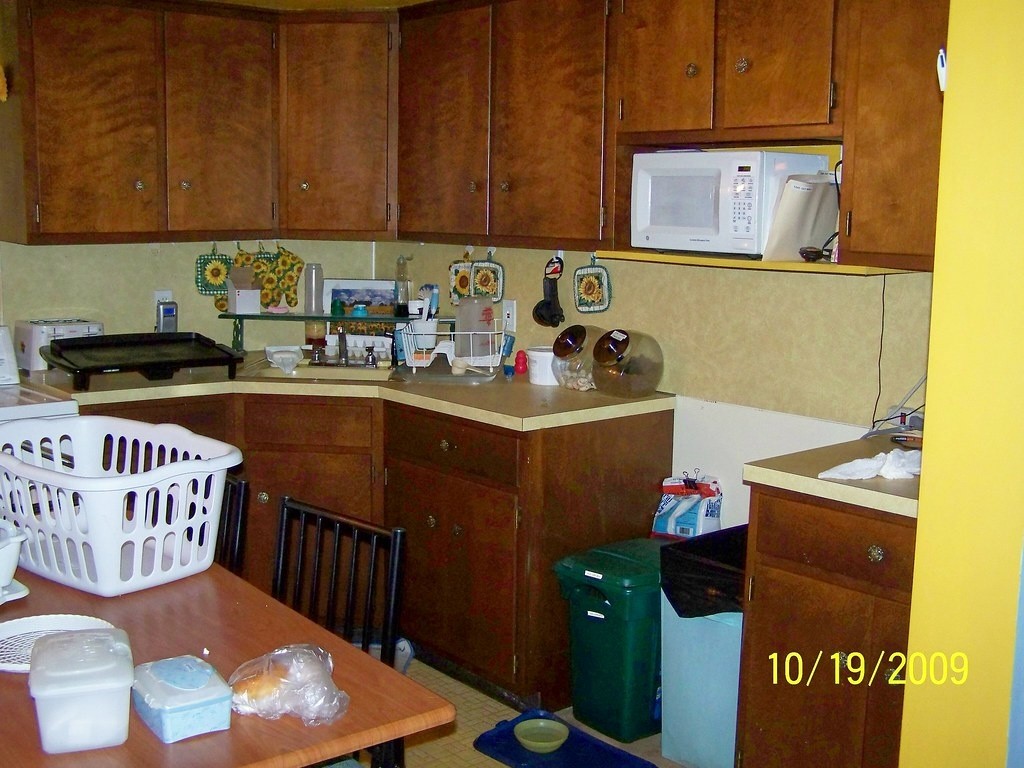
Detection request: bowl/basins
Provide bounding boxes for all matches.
[526,347,560,386]
[513,718,569,753]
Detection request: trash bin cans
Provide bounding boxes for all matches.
[659,523,748,768]
[550,538,662,743]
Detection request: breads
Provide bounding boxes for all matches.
[233,658,290,699]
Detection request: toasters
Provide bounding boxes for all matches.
[13,319,104,373]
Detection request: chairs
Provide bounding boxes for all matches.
[271,494,406,669]
[152,473,249,575]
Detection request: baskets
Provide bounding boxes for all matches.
[399,318,507,369]
[0,415,244,597]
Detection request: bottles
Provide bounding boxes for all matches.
[395,255,409,304]
[304,262,324,315]
[592,328,664,399]
[459,296,495,357]
[551,325,608,391]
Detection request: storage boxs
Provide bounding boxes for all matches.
[225,266,265,315]
[28,628,135,755]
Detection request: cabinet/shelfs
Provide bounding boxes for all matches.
[0,0,949,272]
[733,480,917,768]
[79,393,674,715]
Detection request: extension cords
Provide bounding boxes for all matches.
[888,406,924,430]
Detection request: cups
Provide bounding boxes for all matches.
[412,320,438,349]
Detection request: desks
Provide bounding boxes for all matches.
[0,510,456,768]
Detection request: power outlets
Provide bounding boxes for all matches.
[155,290,172,302]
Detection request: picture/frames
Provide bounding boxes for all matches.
[321,278,410,347]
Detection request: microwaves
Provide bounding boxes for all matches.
[630,150,828,258]
